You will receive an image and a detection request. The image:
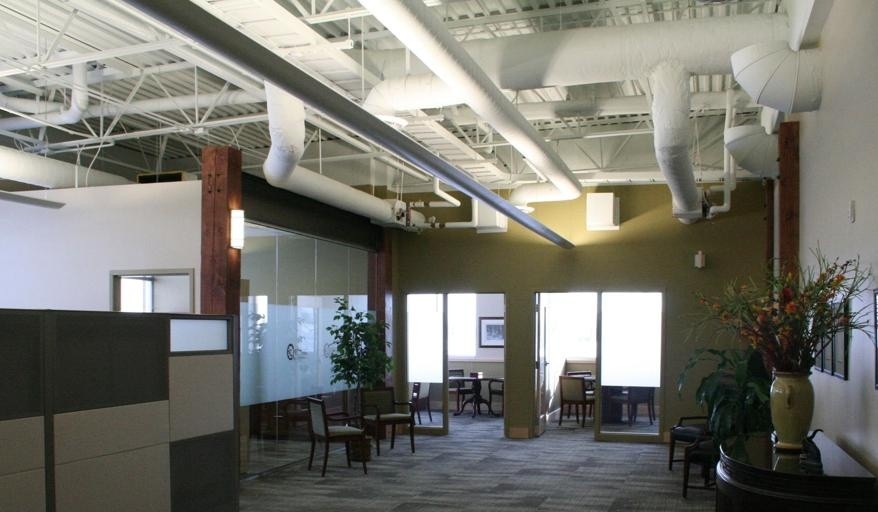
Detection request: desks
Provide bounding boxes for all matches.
[584,377,596,382]
[449,377,504,418]
[714,429,878,512]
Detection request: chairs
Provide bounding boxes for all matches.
[409,382,421,425]
[559,376,596,428]
[489,378,504,416]
[448,369,465,410]
[306,394,368,477]
[567,371,592,418]
[418,382,431,422]
[667,414,708,470]
[682,431,720,498]
[360,387,415,456]
[608,386,656,426]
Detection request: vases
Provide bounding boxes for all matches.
[769,371,815,451]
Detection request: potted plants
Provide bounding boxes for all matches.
[326,296,394,461]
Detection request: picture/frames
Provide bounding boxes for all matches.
[479,317,504,348]
[811,300,848,380]
[872,289,878,390]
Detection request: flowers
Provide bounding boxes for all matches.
[676,240,876,370]
